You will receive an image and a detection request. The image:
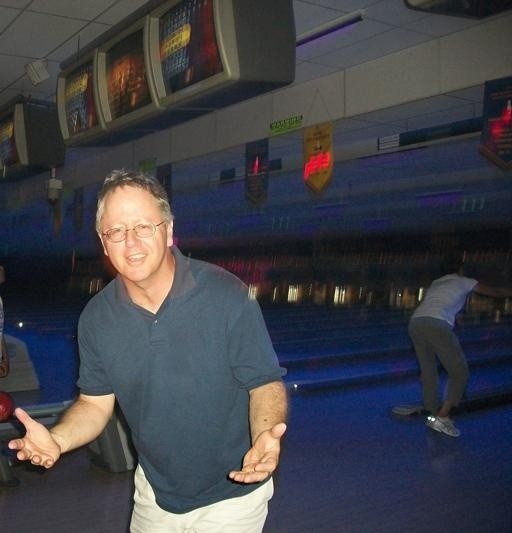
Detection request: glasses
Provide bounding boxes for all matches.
[100,220,167,243]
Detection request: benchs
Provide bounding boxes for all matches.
[0,399,136,488]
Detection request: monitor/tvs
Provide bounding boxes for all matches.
[149,0,296,110]
[1,93,65,186]
[96,2,203,134]
[53,36,157,149]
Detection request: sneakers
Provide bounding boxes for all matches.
[424,413,461,438]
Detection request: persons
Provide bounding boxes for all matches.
[8,170,289,533]
[406,254,512,438]
[0,263,21,486]
